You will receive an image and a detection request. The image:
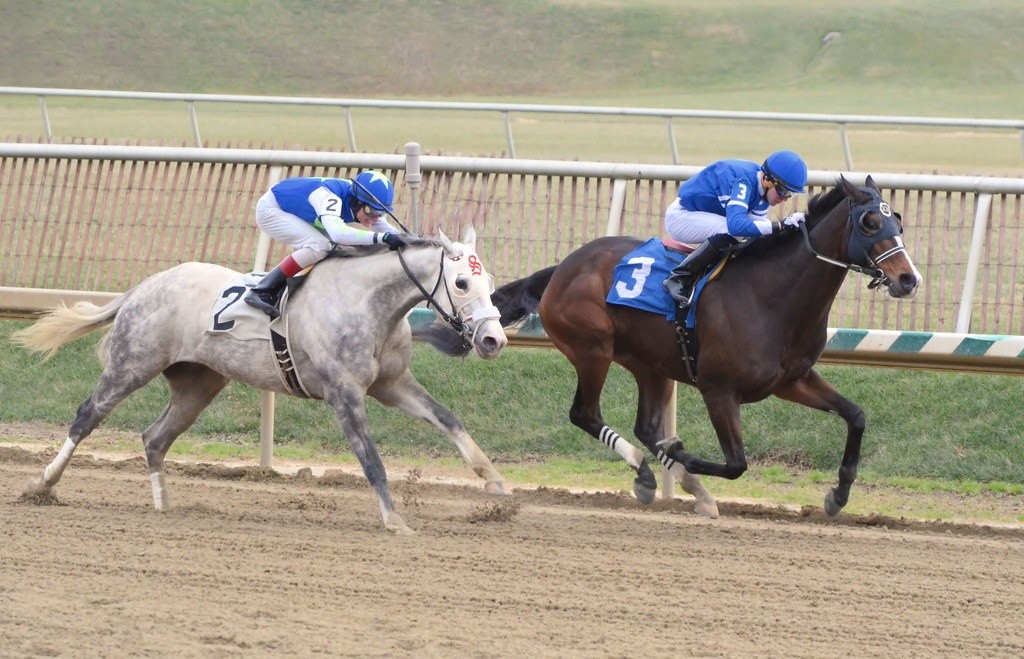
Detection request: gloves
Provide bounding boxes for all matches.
[382,232,408,253]
[772,212,806,234]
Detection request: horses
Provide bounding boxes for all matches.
[8,222,515,535]
[412,173,922,518]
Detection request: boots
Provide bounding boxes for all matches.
[244,265,287,317]
[662,238,721,302]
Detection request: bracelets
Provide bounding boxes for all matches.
[771,221,779,235]
[373,232,379,244]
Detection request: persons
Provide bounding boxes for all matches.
[661,149,807,309]
[243,170,407,316]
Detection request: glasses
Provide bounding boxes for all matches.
[770,179,791,199]
[362,203,385,218]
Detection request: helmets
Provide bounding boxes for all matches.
[760,150,807,193]
[350,170,394,211]
